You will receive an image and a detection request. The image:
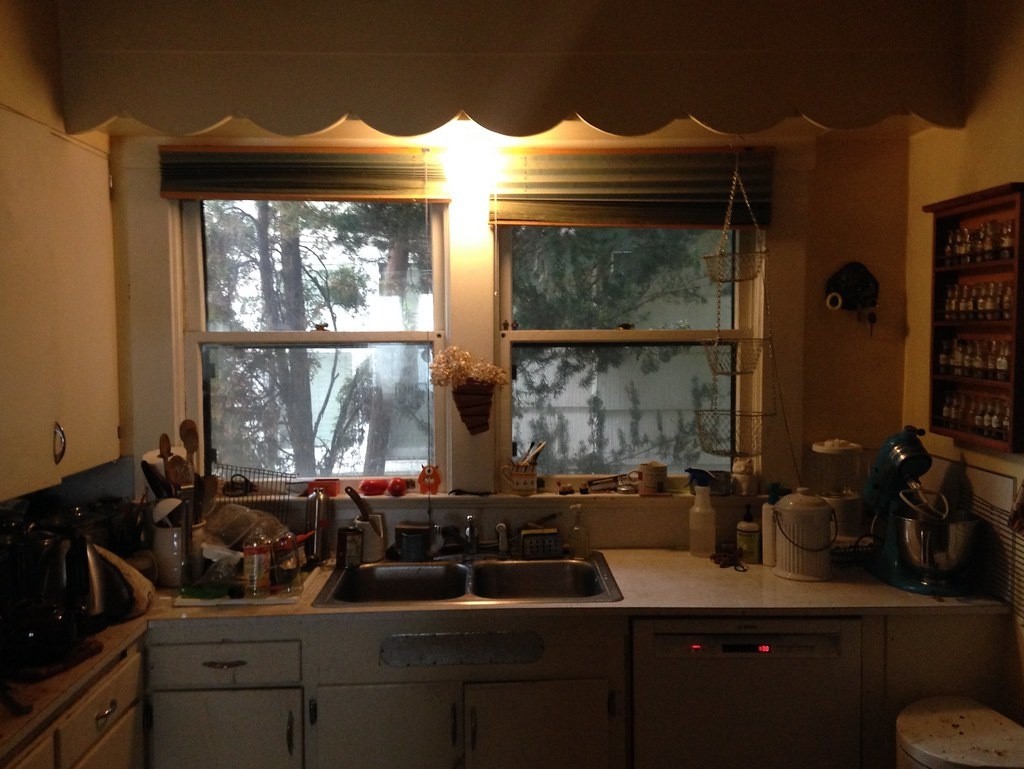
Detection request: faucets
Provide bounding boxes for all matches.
[495,523,508,553]
[465,515,480,548]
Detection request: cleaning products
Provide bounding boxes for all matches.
[682,466,716,558]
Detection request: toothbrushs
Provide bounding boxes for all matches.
[521,441,548,465]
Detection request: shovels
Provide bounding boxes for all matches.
[201,475,220,517]
[160,420,199,493]
[193,472,206,524]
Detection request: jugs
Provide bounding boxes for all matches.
[0,496,160,665]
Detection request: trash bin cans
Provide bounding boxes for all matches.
[896,694,1024,769]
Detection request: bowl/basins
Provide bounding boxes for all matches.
[207,504,285,552]
[688,470,730,496]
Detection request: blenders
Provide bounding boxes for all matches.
[809,438,865,540]
[869,425,979,597]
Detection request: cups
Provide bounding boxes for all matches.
[627,464,667,493]
[401,530,425,563]
[500,463,538,496]
[336,526,364,568]
[152,519,207,587]
[354,514,384,561]
[772,487,836,579]
[730,473,757,496]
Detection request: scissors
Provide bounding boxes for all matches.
[222,474,289,497]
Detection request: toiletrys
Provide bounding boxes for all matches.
[737,504,760,563]
[762,482,782,567]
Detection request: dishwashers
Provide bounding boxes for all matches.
[632,617,863,769]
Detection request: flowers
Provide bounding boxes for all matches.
[429,346,511,393]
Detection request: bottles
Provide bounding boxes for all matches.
[307,488,328,559]
[938,218,1014,265]
[941,281,1012,320]
[242,527,268,596]
[271,526,304,595]
[938,393,1008,442]
[937,337,1010,383]
[736,482,794,567]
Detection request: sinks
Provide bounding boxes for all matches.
[470,561,624,603]
[311,564,466,608]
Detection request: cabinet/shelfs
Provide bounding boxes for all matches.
[1,634,144,769]
[145,622,311,768]
[0,105,123,503]
[311,617,629,769]
[922,183,1024,454]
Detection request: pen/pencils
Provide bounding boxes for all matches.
[507,440,547,465]
